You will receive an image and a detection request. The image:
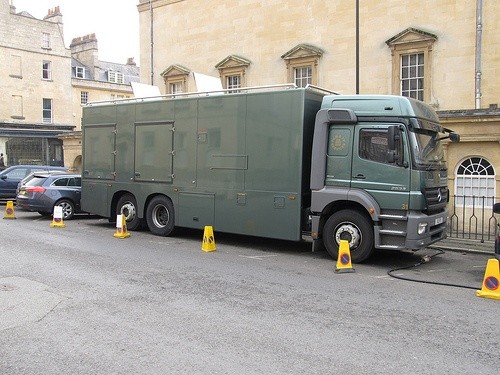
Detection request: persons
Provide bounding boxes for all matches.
[0,153,4,166]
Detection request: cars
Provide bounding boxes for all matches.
[492,202,500,260]
[16,170,69,216]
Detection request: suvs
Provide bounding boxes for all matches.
[16,174,108,221]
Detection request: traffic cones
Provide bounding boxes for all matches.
[201,225,217,253]
[49,206,65,229]
[3,201,17,219]
[334,239,356,274]
[475,258,500,301]
[113,214,131,239]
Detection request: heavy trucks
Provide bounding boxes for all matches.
[79,83,461,264]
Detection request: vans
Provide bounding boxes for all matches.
[0,164,68,207]
